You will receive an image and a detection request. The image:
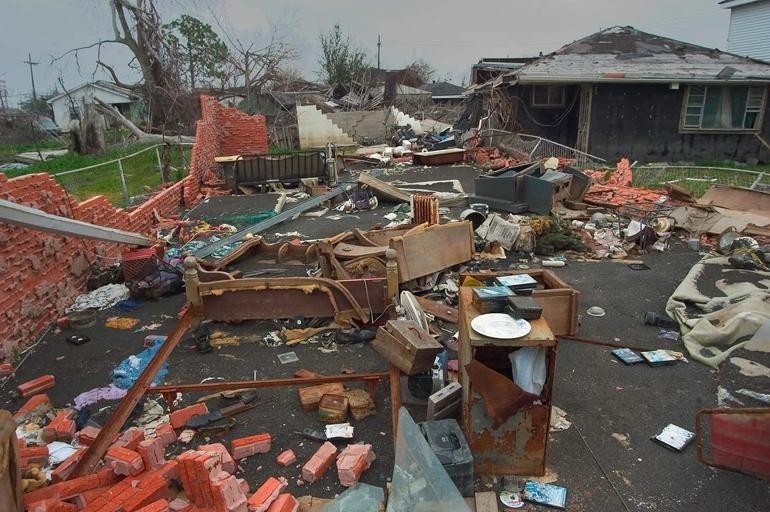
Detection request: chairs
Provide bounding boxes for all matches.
[696,404,769,483]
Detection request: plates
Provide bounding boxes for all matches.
[472,312,532,339]
